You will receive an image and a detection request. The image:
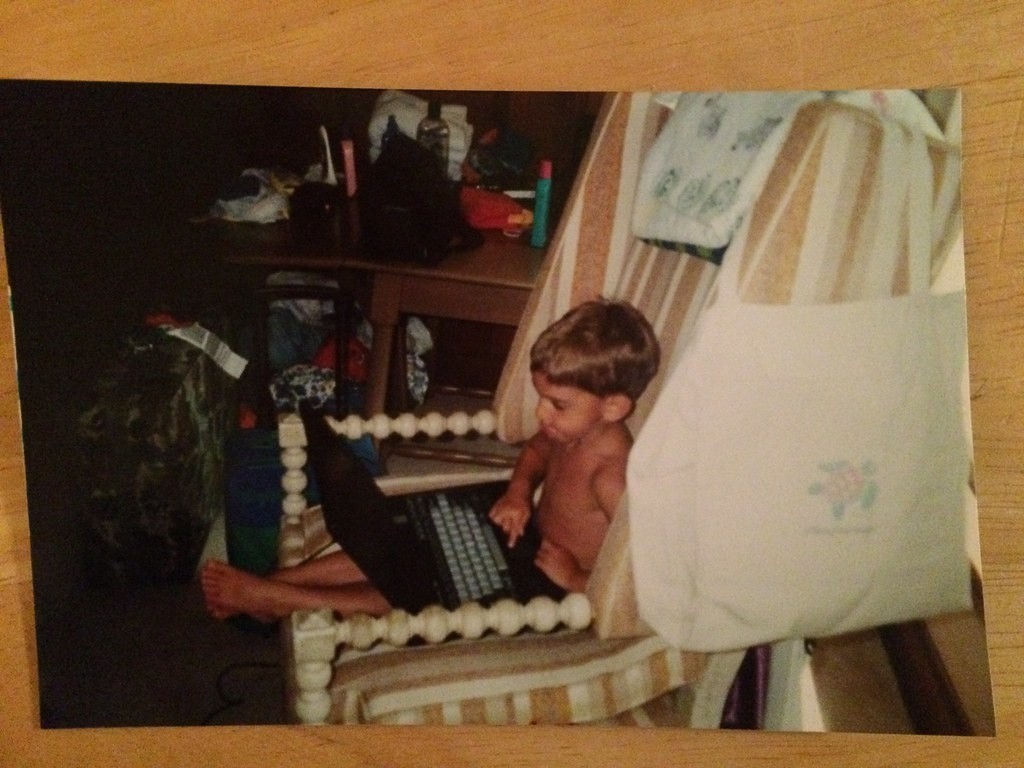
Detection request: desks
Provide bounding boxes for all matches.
[265,204,560,417]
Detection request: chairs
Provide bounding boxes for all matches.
[238,286,370,417]
[276,92,971,725]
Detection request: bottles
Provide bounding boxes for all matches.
[417,101,449,175]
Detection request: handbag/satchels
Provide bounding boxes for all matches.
[625,99,974,654]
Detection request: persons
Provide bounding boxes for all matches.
[202,297,662,633]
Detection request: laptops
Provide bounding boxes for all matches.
[297,399,570,616]
